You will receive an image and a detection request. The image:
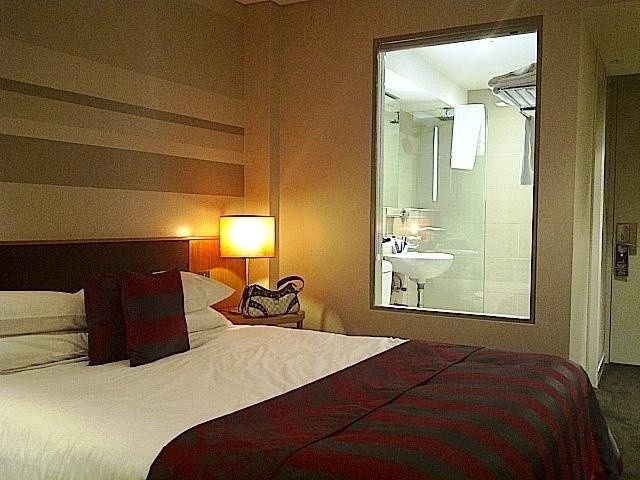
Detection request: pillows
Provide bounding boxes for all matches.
[184,306,229,333]
[119,266,192,368]
[150,268,236,315]
[78,261,153,368]
[0,288,87,335]
[0,330,94,377]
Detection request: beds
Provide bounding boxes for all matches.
[0,238,597,479]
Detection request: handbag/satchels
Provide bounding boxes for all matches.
[238,282,302,319]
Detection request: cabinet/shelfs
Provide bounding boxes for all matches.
[217,305,305,329]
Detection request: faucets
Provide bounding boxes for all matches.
[401,243,419,254]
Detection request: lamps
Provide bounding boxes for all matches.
[217,214,278,314]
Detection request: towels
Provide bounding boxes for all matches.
[450,102,486,171]
[487,60,538,90]
[519,117,536,185]
[491,69,538,118]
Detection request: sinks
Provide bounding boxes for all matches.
[401,252,450,259]
